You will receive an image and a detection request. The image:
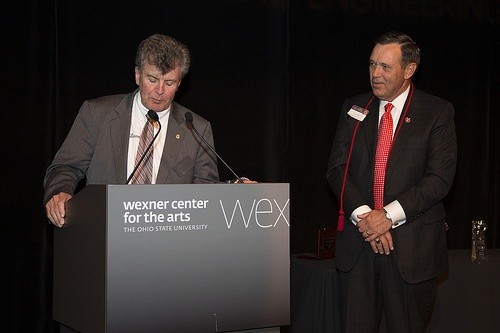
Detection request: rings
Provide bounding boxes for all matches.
[374,239,380,244]
[366,230,370,236]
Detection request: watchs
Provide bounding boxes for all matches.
[383,209,392,221]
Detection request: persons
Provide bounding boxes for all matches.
[43,34,220,228]
[326,31,456,333]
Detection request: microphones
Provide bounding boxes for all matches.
[185,112,244,183]
[125,109,161,184]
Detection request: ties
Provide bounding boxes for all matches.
[131,113,154,185]
[373,102,394,210]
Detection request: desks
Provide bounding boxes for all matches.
[289,250,500,333]
